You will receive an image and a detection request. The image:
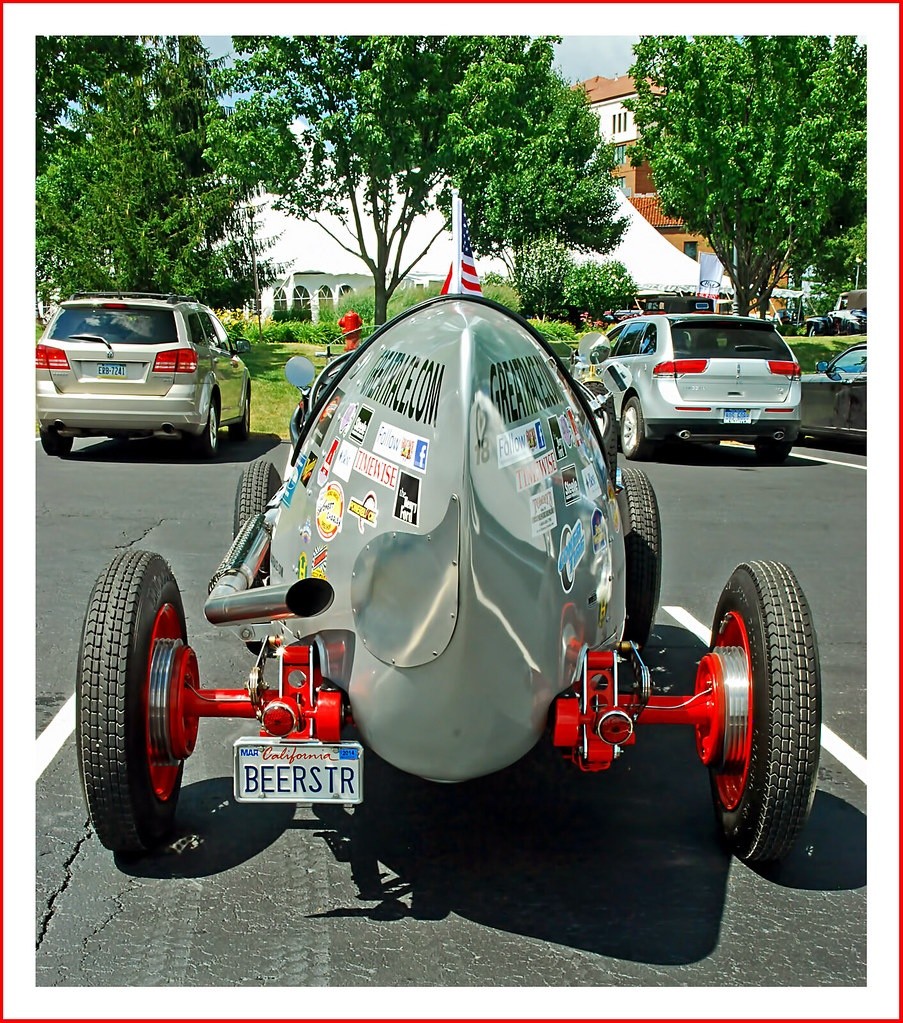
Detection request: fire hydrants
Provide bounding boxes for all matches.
[338,311,363,352]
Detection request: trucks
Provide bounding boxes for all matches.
[834,289,867,314]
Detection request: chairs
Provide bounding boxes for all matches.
[692,331,721,351]
[727,331,761,346]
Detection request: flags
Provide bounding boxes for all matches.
[441,210,483,296]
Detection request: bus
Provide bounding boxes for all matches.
[623,292,715,317]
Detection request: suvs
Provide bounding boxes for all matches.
[573,313,802,465]
[36,292,252,456]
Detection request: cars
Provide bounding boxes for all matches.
[773,309,804,324]
[793,340,866,450]
[805,309,867,336]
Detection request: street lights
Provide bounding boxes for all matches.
[855,255,864,289]
